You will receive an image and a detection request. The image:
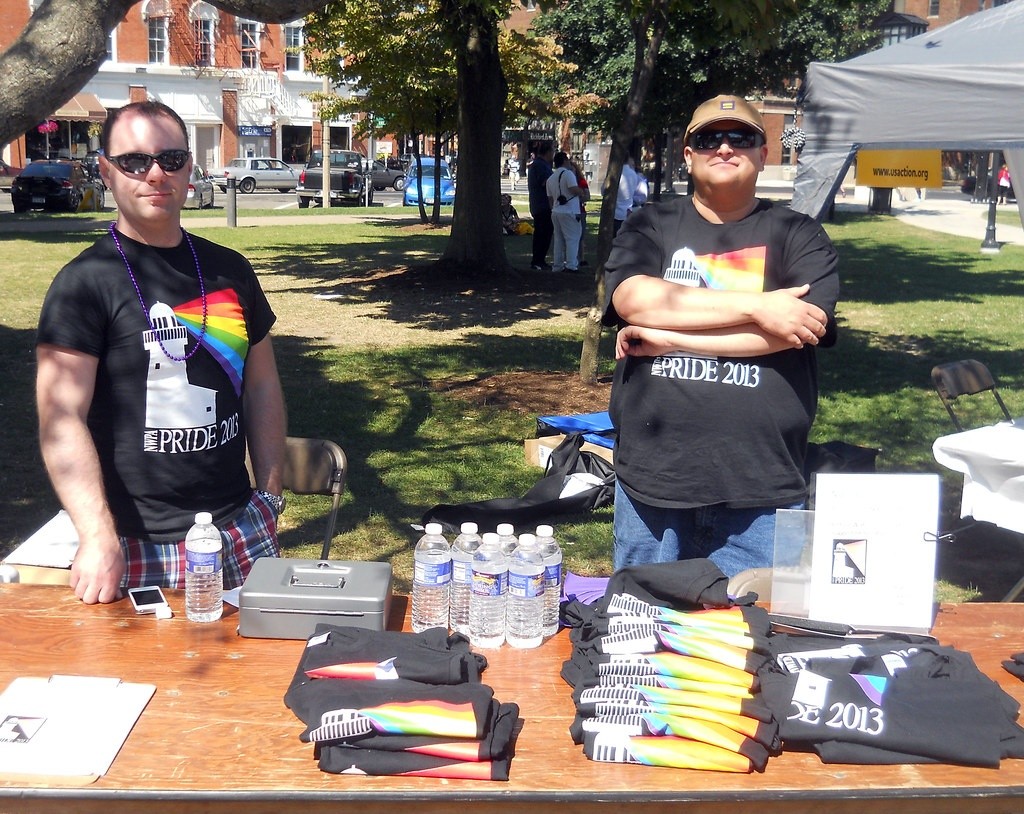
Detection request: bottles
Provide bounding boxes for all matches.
[185,512,224,622]
[451,521,482,630]
[506,533,545,649]
[497,522,520,553]
[412,522,451,634]
[470,532,509,648]
[535,525,562,636]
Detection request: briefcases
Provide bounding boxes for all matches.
[238,557,393,640]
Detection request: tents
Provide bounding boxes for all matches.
[789,0,1024,229]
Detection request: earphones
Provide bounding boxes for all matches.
[685,156,692,173]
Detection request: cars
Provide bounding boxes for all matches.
[11,158,105,213]
[361,159,407,191]
[84,151,111,190]
[181,164,214,209]
[403,157,456,206]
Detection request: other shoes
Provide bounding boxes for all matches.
[566,269,580,274]
[580,260,589,266]
[531,261,552,270]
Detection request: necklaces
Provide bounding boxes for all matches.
[109,223,207,361]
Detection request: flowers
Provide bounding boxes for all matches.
[38,121,58,134]
[87,123,103,139]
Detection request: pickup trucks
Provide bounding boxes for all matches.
[207,158,299,194]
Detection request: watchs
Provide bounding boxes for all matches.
[259,490,286,515]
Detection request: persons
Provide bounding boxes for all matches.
[33,100,292,606]
[500,193,521,236]
[612,151,648,236]
[996,164,1011,206]
[597,92,844,583]
[526,143,591,274]
[507,154,520,191]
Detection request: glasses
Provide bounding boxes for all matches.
[688,129,766,149]
[105,149,192,173]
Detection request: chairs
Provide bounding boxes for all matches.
[931,359,1012,434]
[244,435,347,560]
[251,161,259,170]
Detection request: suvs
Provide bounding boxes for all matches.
[295,150,374,209]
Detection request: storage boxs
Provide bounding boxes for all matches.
[525,434,610,468]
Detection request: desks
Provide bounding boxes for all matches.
[933,416,1024,602]
[0,581,1024,814]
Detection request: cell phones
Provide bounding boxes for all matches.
[128,586,169,614]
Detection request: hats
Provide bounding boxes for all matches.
[683,94,767,146]
